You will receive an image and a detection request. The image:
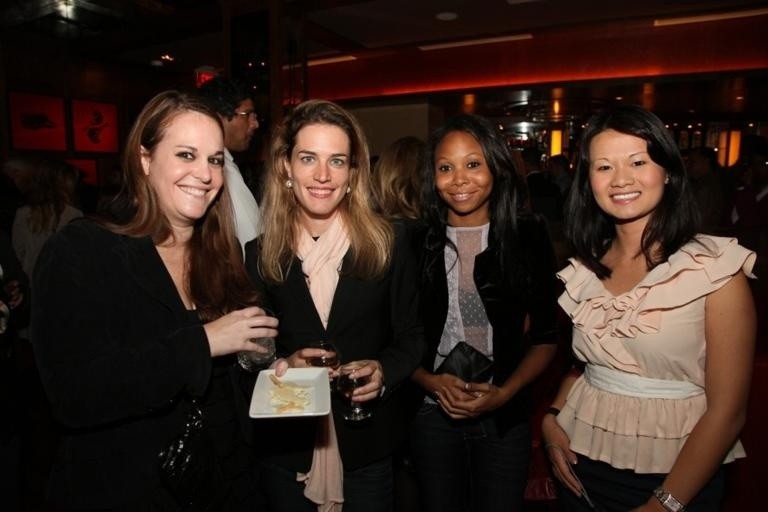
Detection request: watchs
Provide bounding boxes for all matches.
[652,486,684,512]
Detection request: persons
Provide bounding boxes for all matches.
[87,163,130,213]
[403,112,566,512]
[28,89,279,512]
[1,171,30,445]
[515,141,563,225]
[684,146,728,234]
[193,69,264,290]
[544,154,574,188]
[728,161,767,260]
[369,136,433,246]
[541,103,757,512]
[244,99,422,512]
[12,160,85,294]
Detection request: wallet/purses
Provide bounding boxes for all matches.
[429,341,497,412]
